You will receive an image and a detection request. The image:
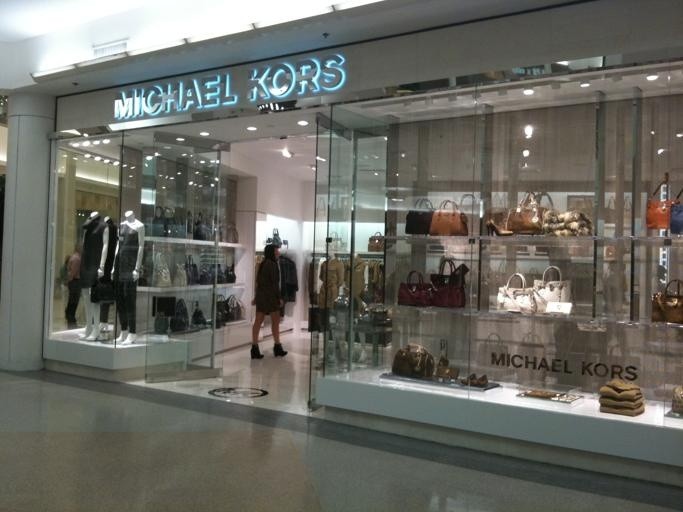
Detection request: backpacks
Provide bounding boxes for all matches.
[392,342,436,380]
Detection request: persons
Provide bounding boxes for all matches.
[80,212,100,340]
[250,245,288,358]
[65,240,82,326]
[99,217,118,323]
[111,210,145,345]
[60,255,70,312]
[344,249,368,362]
[319,241,344,309]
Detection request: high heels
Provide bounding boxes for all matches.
[274,344,288,357]
[251,345,264,359]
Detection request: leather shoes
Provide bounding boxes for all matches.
[470,375,488,385]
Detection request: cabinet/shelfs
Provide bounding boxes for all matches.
[134,235,248,334]
[372,232,682,402]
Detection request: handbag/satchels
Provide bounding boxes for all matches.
[651,279,683,324]
[435,364,460,379]
[398,190,578,315]
[646,182,680,227]
[477,333,641,386]
[90,276,114,302]
[670,188,683,235]
[326,232,342,250]
[274,234,282,248]
[368,232,385,252]
[145,216,241,332]
